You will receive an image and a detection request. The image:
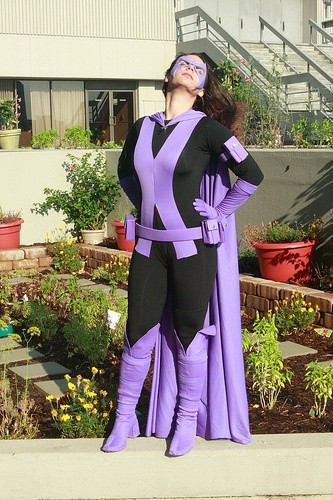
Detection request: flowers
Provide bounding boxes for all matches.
[240,214,324,248]
[30,149,123,230]
[0,95,22,129]
[212,59,258,105]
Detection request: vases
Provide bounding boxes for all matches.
[0,128,22,149]
[207,101,248,141]
[251,238,317,285]
[79,227,105,244]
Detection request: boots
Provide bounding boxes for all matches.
[100,323,161,452]
[167,324,217,458]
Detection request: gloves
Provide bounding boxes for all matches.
[193,178,260,223]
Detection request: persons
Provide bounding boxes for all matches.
[274,126,283,146]
[102,51,264,456]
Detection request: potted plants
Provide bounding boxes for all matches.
[111,200,139,252]
[0,207,25,248]
